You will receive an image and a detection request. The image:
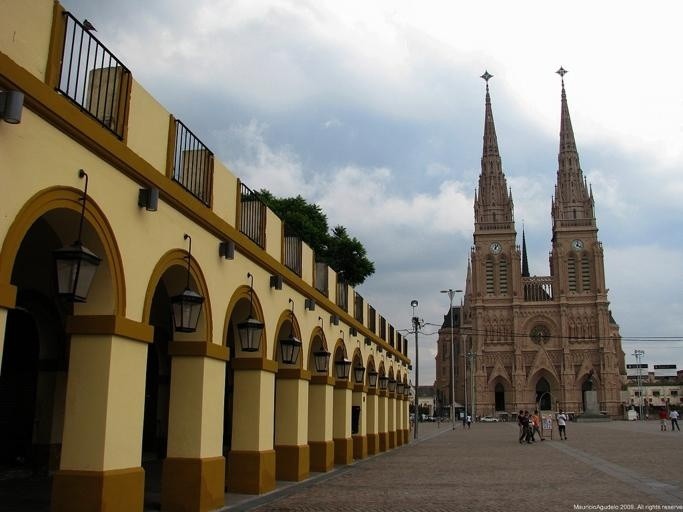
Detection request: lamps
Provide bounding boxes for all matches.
[313,315,412,397]
[279,298,301,364]
[236,272,265,351]
[270,274,283,290]
[219,241,234,260]
[138,188,159,211]
[170,232,205,334]
[0,88,25,125]
[304,298,316,311]
[52,169,103,307]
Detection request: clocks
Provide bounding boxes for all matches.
[489,241,503,255]
[571,239,584,252]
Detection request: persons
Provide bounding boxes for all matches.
[517,410,545,444]
[556,409,567,440]
[659,407,680,432]
[466,413,472,428]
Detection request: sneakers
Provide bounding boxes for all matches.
[541,439,545,441]
[519,439,535,444]
[561,437,568,440]
[660,429,666,431]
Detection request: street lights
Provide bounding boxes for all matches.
[632,349,644,420]
[440,289,463,428]
[464,352,471,424]
[409,300,419,439]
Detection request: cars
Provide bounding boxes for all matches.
[480,416,498,423]
[409,413,436,422]
